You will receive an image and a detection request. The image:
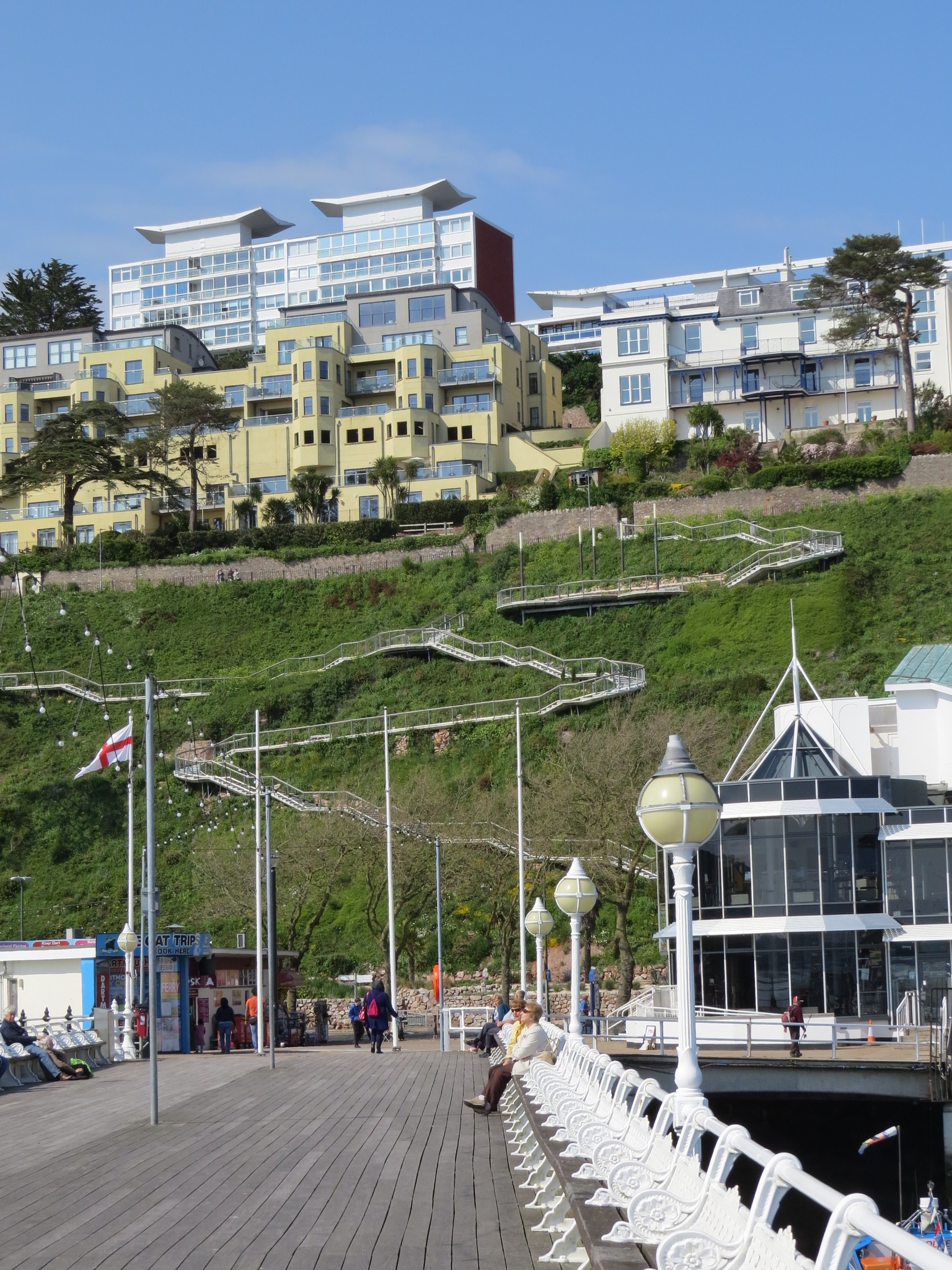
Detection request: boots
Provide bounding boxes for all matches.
[376,1047,383,1053]
[370,1041,375,1053]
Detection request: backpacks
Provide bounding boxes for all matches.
[366,989,385,1017]
[781,1006,799,1027]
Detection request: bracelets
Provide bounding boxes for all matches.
[245,1020,247,1021]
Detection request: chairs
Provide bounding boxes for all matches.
[484,1016,952,1270]
[0,1017,113,1091]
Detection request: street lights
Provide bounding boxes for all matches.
[117,923,139,1061]
[525,897,554,1019]
[555,858,598,1043]
[636,733,724,1168]
[10,876,31,941]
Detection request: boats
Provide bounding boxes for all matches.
[844,1180,952,1270]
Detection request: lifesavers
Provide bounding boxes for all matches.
[433,964,439,1002]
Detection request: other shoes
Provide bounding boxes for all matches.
[226,1050,231,1053]
[200,1052,203,1054]
[219,1050,225,1054]
[355,1045,361,1048]
[0,1088,5,1092]
[790,1054,800,1058]
[193,1051,198,1054]
[797,1053,803,1057]
[254,1051,257,1054]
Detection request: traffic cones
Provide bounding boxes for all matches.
[862,1019,881,1047]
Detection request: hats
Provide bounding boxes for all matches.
[792,996,804,1002]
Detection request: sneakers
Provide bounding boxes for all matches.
[463,1096,486,1109]
[472,1109,491,1116]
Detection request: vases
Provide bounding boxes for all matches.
[749,429,754,433]
[871,416,877,421]
[855,419,859,423]
[786,426,790,429]
[839,420,844,424]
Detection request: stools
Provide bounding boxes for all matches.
[860,869,877,890]
[887,875,902,899]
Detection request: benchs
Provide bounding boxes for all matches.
[695,981,706,1010]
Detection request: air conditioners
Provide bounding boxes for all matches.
[66,929,84,939]
[237,934,247,948]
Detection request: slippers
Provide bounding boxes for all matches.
[465,1048,477,1054]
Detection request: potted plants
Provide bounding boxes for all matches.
[386,317,394,324]
[694,433,698,438]
[823,419,829,425]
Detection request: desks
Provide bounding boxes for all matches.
[708,984,723,1007]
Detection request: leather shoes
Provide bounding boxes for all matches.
[464,1040,477,1047]
[58,1072,72,1081]
[479,1052,490,1058]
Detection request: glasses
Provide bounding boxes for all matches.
[511,1008,521,1013]
[521,1008,535,1014]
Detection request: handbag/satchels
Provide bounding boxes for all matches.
[359,1004,367,1023]
[541,1037,559,1065]
[249,1016,257,1025]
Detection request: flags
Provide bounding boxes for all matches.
[75,722,132,779]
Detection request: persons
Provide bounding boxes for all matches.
[348,998,371,1048]
[363,980,398,1053]
[194,1018,206,1053]
[228,568,239,582]
[0,1006,73,1080]
[0,1055,9,1091]
[216,568,225,583]
[488,999,527,1111]
[245,988,267,1053]
[39,1036,84,1081]
[463,1002,552,1116]
[216,997,234,1053]
[465,994,511,1054]
[580,995,590,1016]
[464,990,525,1057]
[784,996,806,1057]
